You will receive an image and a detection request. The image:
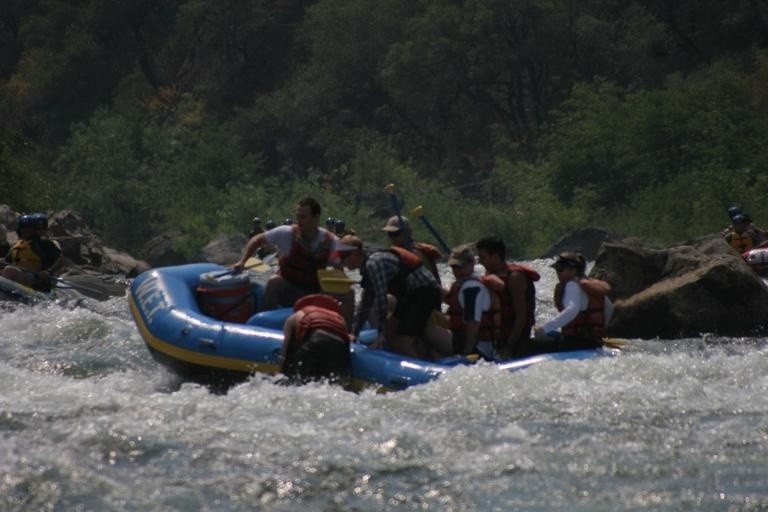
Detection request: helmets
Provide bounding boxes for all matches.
[266,220,276,230]
[282,218,293,225]
[252,217,261,224]
[17,212,49,229]
[727,206,744,224]
[325,216,346,227]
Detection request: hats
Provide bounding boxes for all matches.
[382,215,409,232]
[549,254,576,271]
[448,245,474,266]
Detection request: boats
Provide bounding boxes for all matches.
[127,262,624,395]
[0,276,85,306]
[742,246,768,270]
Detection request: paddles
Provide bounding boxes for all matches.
[543,329,632,348]
[317,268,362,294]
[51,284,126,297]
[206,256,272,281]
[51,274,103,283]
[0,260,109,301]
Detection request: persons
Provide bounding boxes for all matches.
[231,196,542,359]
[720,207,768,252]
[526,252,616,355]
[280,294,352,384]
[0,213,64,293]
